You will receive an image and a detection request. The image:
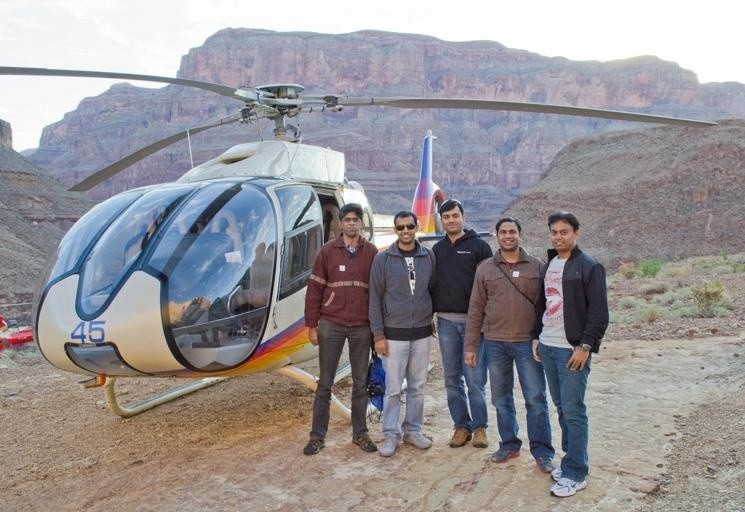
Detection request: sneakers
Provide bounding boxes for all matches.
[473,428,488,448]
[403,434,431,448]
[304,440,325,454]
[450,428,471,447]
[379,438,399,456]
[491,448,520,463]
[536,456,590,496]
[352,436,377,452]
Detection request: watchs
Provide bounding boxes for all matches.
[579,343,592,352]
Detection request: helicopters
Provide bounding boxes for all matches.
[0,66,719,425]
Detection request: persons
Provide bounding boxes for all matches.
[528,210,609,498]
[368,211,436,458]
[141,218,158,250]
[431,199,493,448]
[266,243,273,257]
[303,204,378,455]
[463,217,556,473]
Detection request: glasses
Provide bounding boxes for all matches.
[396,224,416,230]
